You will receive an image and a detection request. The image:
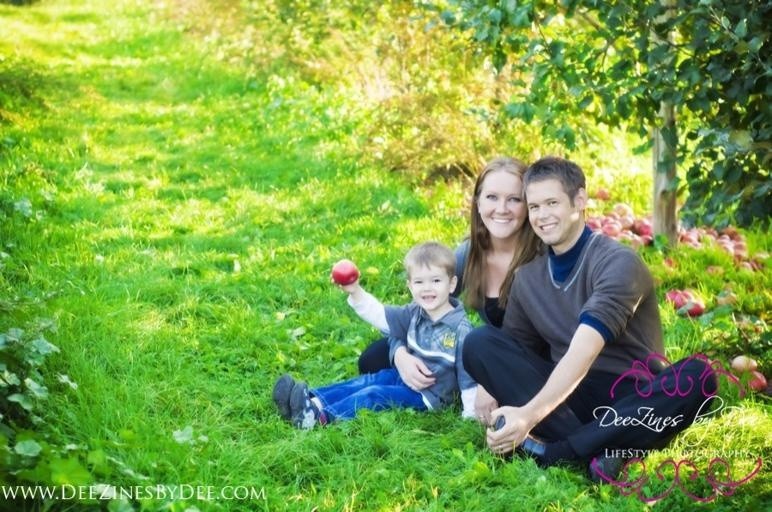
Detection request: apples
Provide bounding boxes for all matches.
[586,187,772,391]
[332,258,359,286]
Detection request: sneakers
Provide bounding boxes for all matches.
[271,375,329,432]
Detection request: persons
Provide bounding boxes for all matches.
[461,155,722,488]
[270,238,481,433]
[450,156,547,328]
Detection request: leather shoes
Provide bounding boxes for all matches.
[495,414,547,469]
[589,446,632,486]
